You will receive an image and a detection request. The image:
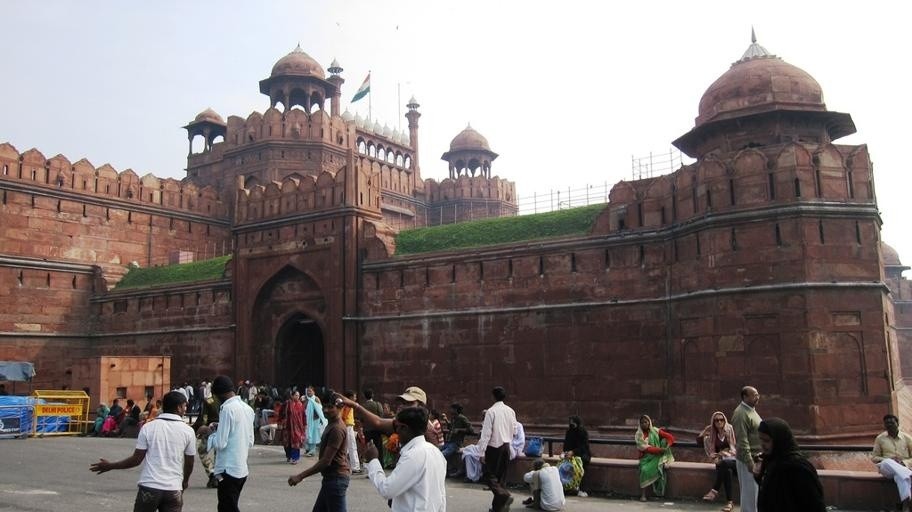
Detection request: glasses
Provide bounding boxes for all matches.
[395,420,406,426]
[713,419,724,422]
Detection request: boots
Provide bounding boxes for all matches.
[447,464,465,478]
[464,477,479,483]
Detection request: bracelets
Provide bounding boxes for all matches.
[355,402,359,411]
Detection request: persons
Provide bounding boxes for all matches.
[363,405,448,512]
[91,393,163,438]
[341,387,444,478]
[170,380,257,439]
[90,392,195,512]
[523,415,591,510]
[699,386,825,511]
[634,415,675,502]
[206,374,256,512]
[287,392,351,512]
[334,386,446,448]
[873,411,912,510]
[448,385,525,510]
[257,384,331,446]
[192,381,218,488]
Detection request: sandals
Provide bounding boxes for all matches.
[291,459,297,464]
[304,450,312,457]
[722,503,734,512]
[702,491,718,503]
[287,459,291,463]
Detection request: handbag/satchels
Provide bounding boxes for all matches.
[525,437,544,456]
[274,424,289,445]
[556,455,585,496]
[533,471,541,510]
[559,462,573,484]
[364,425,383,440]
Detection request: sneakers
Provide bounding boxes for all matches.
[207,473,218,488]
[501,496,513,512]
[577,491,588,497]
[526,502,535,508]
[523,498,532,504]
[903,499,910,512]
[352,470,366,475]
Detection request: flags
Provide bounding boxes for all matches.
[351,71,371,103]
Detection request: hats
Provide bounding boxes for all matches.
[213,376,236,394]
[396,386,427,405]
